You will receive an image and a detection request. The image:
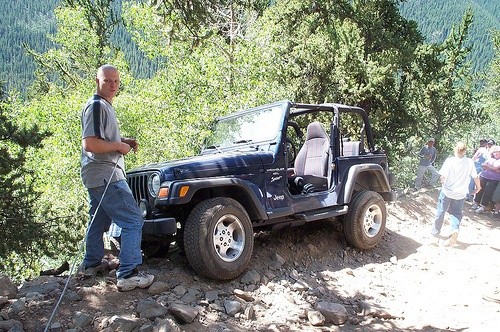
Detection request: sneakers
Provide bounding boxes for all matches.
[466,196,500,214]
[84,259,108,276]
[117,272,154,291]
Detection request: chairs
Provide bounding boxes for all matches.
[293,121,333,187]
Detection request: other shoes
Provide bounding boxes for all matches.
[449,236,457,243]
[431,237,438,245]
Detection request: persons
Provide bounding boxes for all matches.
[81,64,155,292]
[431,141,482,246]
[465,139,500,215]
[414,138,440,191]
